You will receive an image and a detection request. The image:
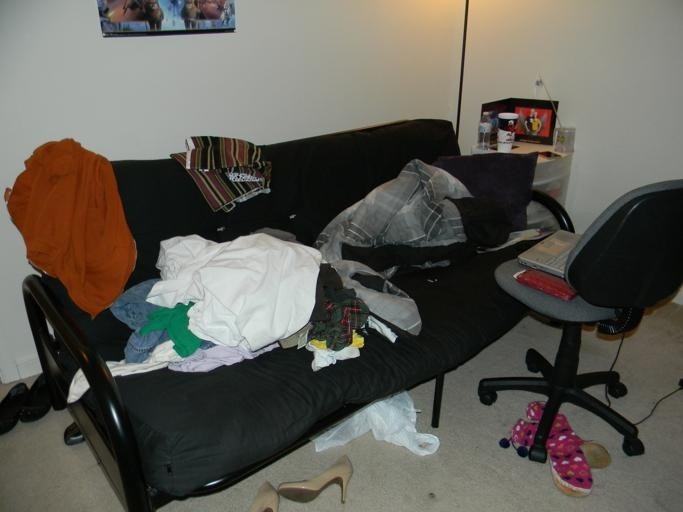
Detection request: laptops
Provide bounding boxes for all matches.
[517,230,583,278]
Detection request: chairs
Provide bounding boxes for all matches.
[478,182,681,463]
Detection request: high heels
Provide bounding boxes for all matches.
[277,455,354,503]
[246,482,279,511]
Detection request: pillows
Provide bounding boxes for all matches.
[431,153,537,231]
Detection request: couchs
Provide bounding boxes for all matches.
[23,118,575,511]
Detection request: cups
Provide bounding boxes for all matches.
[496,112,520,152]
[555,128,575,153]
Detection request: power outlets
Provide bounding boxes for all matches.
[527,75,546,91]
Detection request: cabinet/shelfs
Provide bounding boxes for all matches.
[469,125,579,228]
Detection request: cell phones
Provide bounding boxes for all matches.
[538,151,560,160]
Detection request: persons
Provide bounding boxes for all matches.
[522,108,542,137]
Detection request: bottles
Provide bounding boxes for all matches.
[476,112,492,150]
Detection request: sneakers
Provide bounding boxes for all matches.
[19,373,55,421]
[0,382,28,435]
